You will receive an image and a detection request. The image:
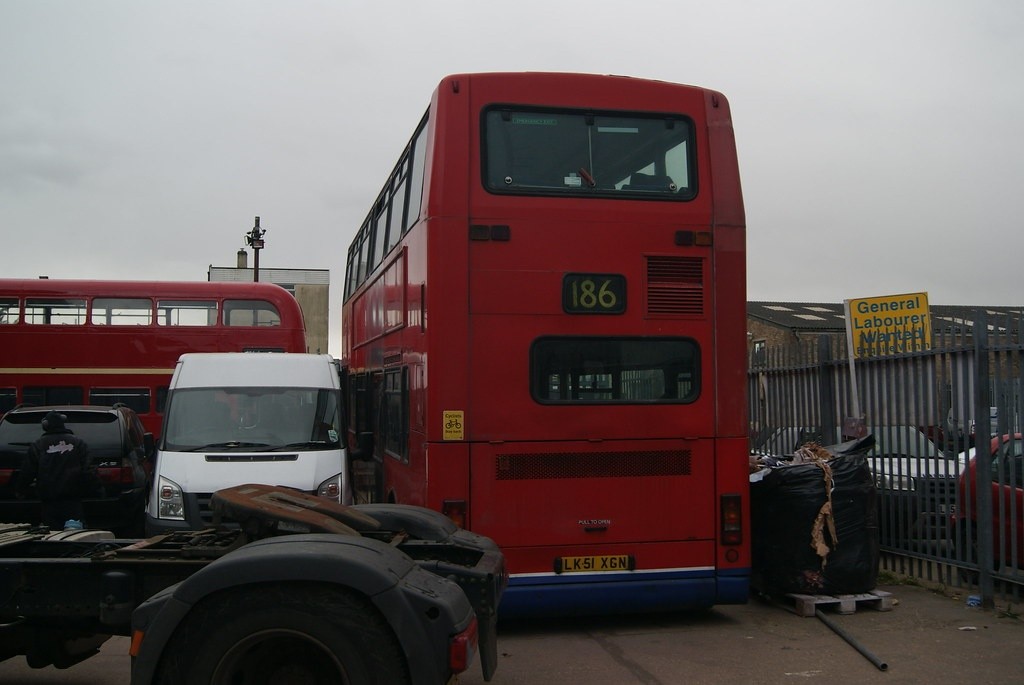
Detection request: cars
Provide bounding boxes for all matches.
[954,433,1024,572]
[752,425,984,545]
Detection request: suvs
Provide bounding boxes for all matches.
[0,401,155,534]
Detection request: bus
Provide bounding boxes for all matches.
[343,73,757,612]
[0,273,312,437]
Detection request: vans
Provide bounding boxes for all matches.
[147,353,352,538]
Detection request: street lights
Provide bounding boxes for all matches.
[234,218,267,326]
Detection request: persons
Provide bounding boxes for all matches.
[16,411,88,531]
